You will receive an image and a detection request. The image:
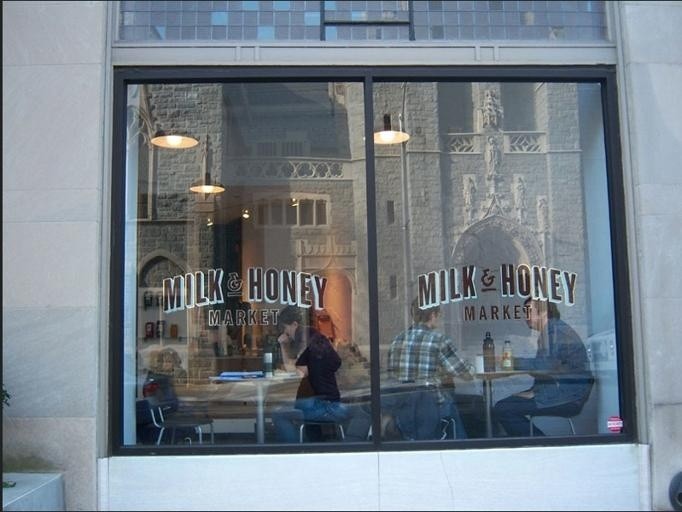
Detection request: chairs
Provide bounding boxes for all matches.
[526,374,597,437]
[382,383,460,444]
[143,367,215,447]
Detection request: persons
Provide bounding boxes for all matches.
[270,304,357,443]
[331,338,373,440]
[226,302,252,356]
[387,296,477,440]
[491,297,595,436]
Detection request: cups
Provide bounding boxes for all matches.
[475,354,484,374]
[145,321,178,338]
[144,291,163,306]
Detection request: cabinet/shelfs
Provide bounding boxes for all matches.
[135,282,194,398]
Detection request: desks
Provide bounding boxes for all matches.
[456,370,556,441]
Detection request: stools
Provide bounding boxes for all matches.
[292,419,353,447]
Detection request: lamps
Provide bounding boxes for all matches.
[187,178,227,195]
[150,128,200,150]
[364,125,413,147]
[200,188,300,229]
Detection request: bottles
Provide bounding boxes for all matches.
[263,343,276,378]
[483,332,496,372]
[502,340,512,370]
